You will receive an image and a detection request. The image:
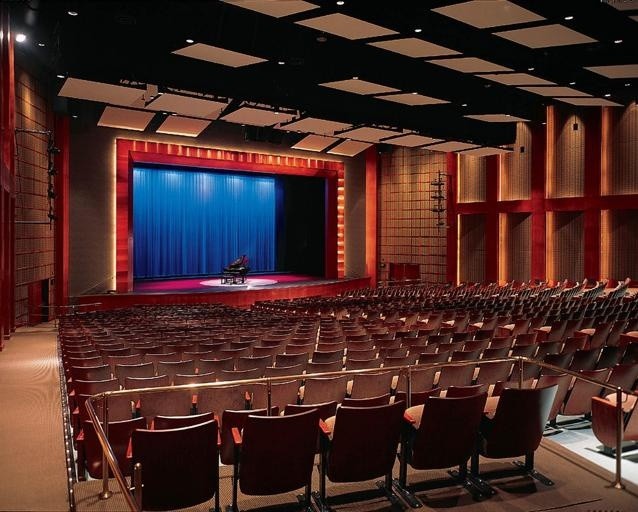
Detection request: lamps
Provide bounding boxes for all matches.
[46,146,61,222]
[431,182,445,227]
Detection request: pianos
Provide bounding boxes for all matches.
[222,255,251,283]
